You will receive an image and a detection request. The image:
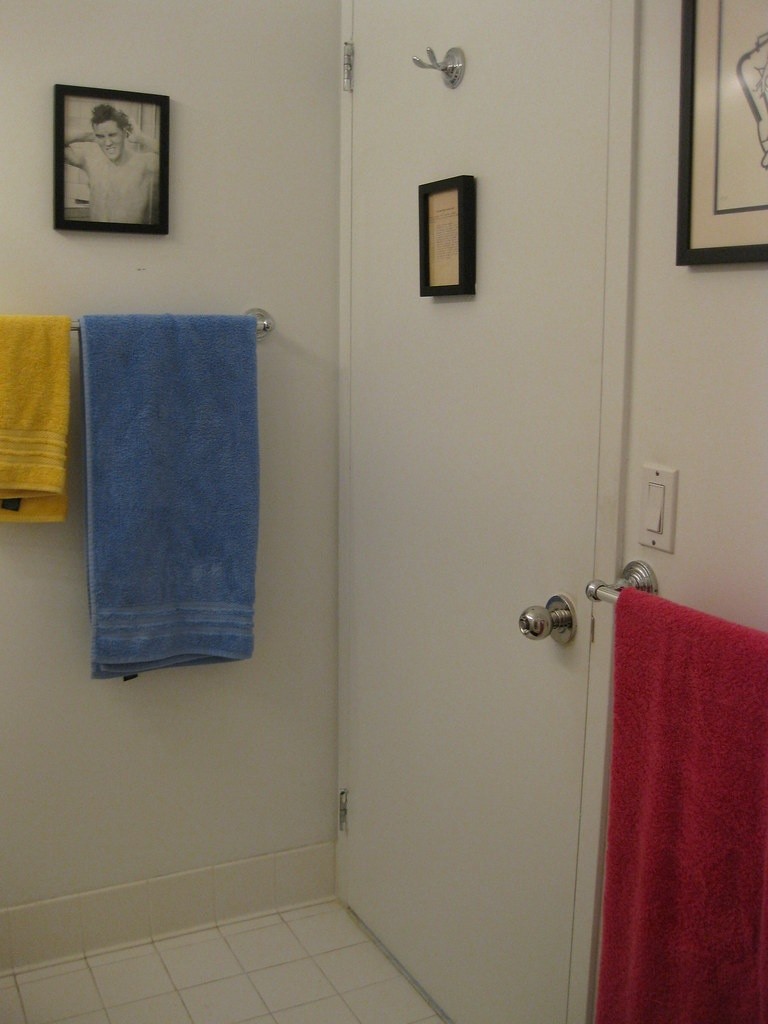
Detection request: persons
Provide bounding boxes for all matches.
[60,103,163,222]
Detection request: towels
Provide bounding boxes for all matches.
[79,313,261,681]
[0,314,72,524]
[592,586,768,1024]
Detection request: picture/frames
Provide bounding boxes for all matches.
[53,83,170,236]
[677,0,768,267]
[415,175,476,297]
